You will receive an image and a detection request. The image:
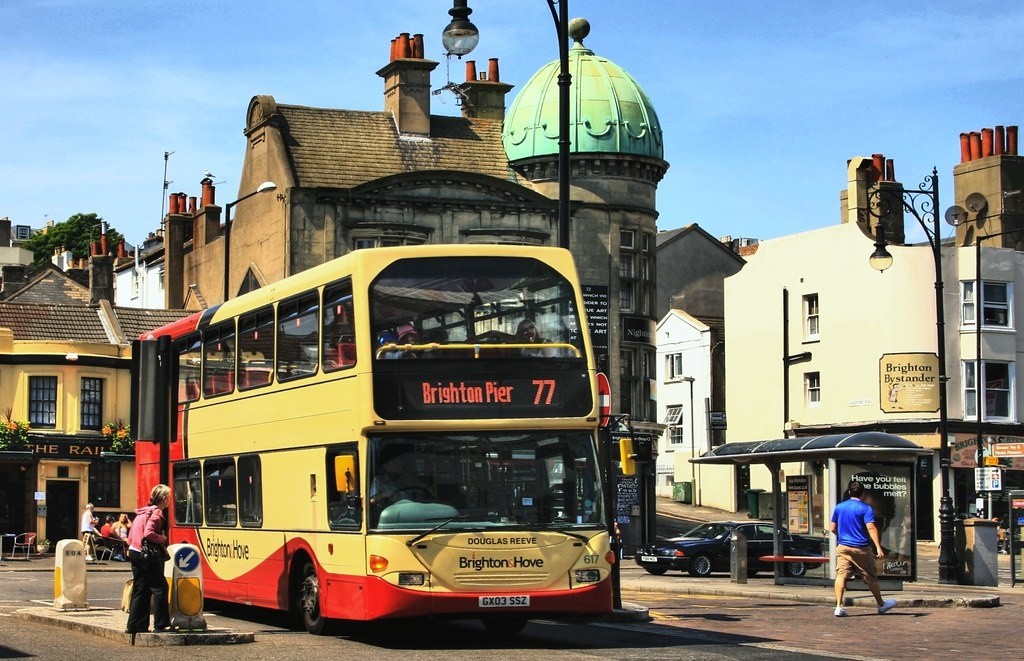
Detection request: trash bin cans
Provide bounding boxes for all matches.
[744,488,766,519]
[954,516,999,587]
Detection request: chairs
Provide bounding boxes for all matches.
[186,334,356,397]
[94,535,127,561]
[11,531,37,560]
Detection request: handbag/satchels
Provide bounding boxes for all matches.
[141,536,171,563]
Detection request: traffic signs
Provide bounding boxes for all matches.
[974,467,1002,492]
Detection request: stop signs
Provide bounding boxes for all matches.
[596,370,611,430]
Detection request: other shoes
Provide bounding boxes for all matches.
[86,555,93,560]
[114,554,124,562]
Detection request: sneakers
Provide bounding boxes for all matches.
[877,599,896,614]
[834,606,848,617]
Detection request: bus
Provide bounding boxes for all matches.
[130,244,640,637]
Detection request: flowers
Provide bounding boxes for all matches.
[0,408,29,449]
[101,419,135,454]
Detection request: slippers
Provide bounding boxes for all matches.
[125,629,151,633]
[153,626,179,633]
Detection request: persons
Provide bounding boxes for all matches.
[517,320,554,357]
[82,503,99,560]
[124,484,178,633]
[93,513,132,561]
[849,472,907,572]
[992,512,1009,552]
[371,457,434,503]
[831,481,896,616]
[382,325,439,359]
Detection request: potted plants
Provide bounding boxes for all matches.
[37,539,51,553]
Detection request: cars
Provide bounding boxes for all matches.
[633,521,823,578]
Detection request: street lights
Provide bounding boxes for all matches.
[441,0,573,359]
[223,181,278,362]
[682,377,696,507]
[865,163,961,587]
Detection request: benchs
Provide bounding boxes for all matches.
[760,554,829,563]
